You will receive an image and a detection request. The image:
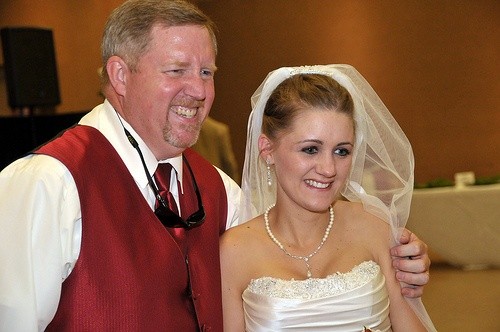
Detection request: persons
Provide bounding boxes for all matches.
[193,113,238,186]
[0,0,433,331]
[219,64,438,332]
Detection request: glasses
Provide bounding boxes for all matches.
[122,128,205,230]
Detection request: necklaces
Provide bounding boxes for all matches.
[264,201,335,280]
